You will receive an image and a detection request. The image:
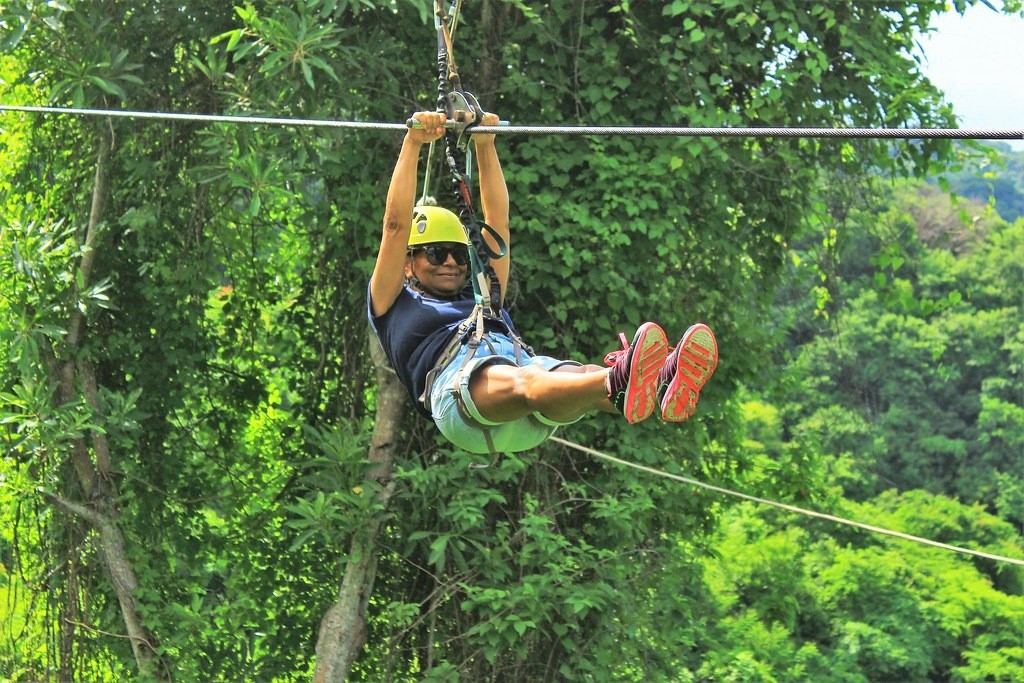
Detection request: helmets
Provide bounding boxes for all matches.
[408,205,471,247]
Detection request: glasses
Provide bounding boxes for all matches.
[414,243,469,266]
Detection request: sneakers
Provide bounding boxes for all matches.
[604,322,669,423]
[656,323,718,422]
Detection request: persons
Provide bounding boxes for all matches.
[364,111,720,455]
